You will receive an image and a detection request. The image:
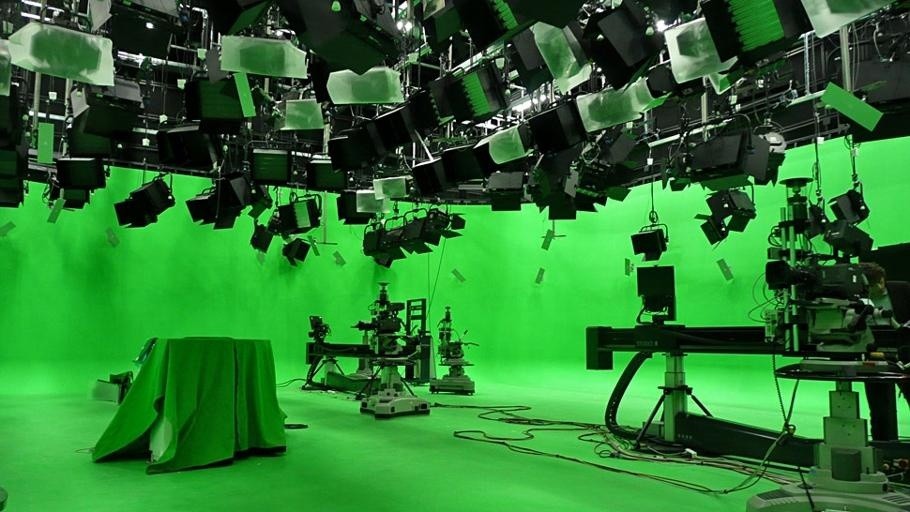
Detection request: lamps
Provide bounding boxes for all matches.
[0,0,875,267]
